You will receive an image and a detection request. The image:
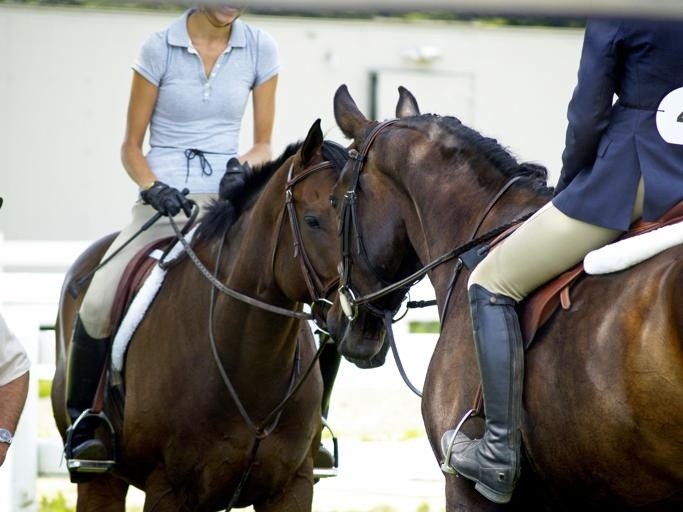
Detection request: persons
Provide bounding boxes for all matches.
[0,316,30,471]
[440,16,683,504]
[64,1,280,483]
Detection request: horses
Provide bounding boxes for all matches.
[49,117,390,512]
[325,82,683,512]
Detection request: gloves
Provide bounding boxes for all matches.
[219,158,246,196]
[140,180,193,218]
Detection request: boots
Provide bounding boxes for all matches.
[64,312,114,485]
[438,283,525,505]
[310,342,341,483]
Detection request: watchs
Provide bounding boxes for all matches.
[0,427,12,445]
